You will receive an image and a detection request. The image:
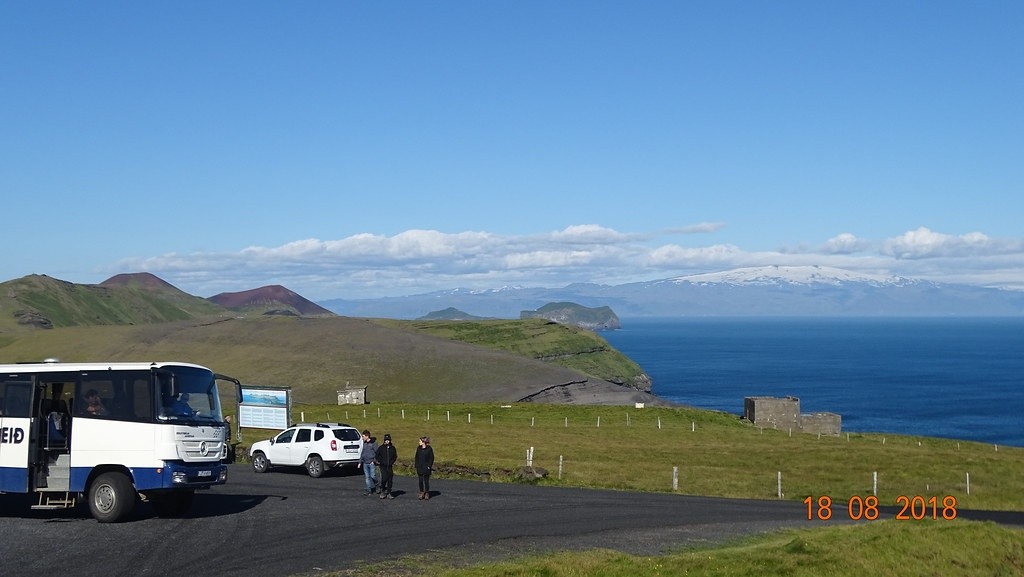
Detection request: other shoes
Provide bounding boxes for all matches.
[376,485,380,493]
[363,492,373,496]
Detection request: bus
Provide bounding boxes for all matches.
[0,357,243,524]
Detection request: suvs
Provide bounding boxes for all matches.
[249,422,363,479]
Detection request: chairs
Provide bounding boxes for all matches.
[41,397,87,439]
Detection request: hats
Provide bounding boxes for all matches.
[384,434,391,440]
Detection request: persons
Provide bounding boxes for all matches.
[375,434,398,499]
[414,436,435,500]
[81,389,106,416]
[358,429,381,496]
[172,393,195,416]
[223,414,233,464]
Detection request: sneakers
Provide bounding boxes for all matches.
[379,493,395,499]
[418,492,429,500]
